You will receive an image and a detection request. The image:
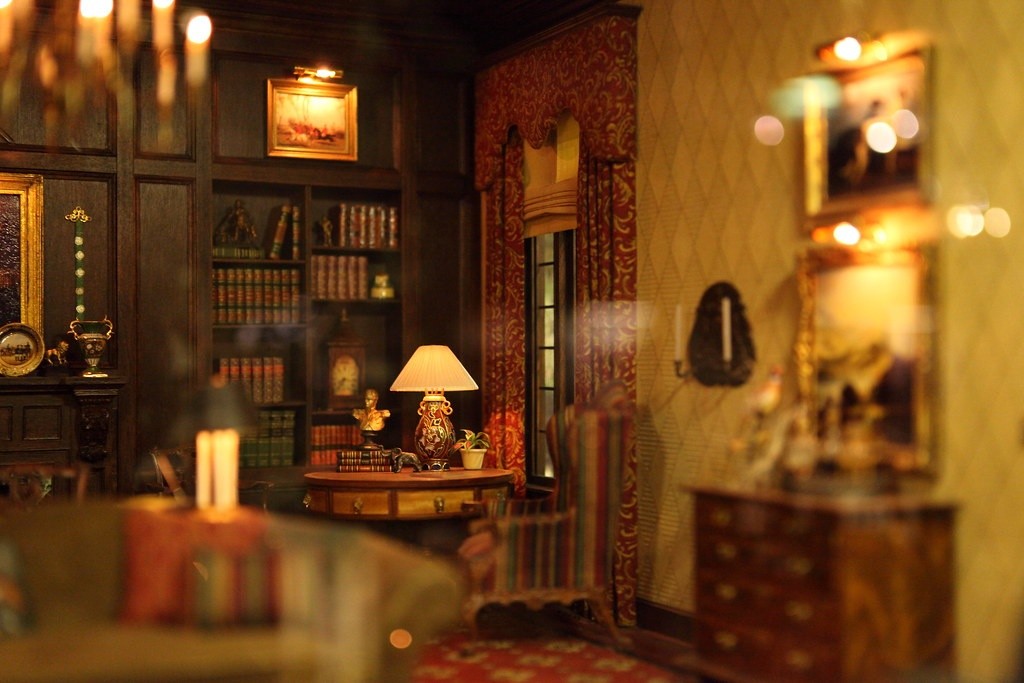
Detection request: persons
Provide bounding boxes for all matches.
[317,214,335,248]
[216,198,258,250]
[13,342,31,359]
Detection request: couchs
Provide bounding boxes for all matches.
[474,400,624,633]
[1,493,467,683]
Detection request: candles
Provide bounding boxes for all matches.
[719,295,734,363]
[673,286,686,361]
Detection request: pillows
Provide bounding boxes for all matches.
[1,503,142,631]
[181,539,286,627]
[119,503,274,627]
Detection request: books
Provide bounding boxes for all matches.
[213,245,267,260]
[264,205,290,258]
[337,457,391,465]
[281,204,303,261]
[211,270,303,325]
[310,254,370,301]
[312,424,366,465]
[332,201,398,248]
[336,464,392,472]
[335,450,390,458]
[215,355,296,467]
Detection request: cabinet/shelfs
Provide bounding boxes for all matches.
[687,482,962,683]
[0,371,128,505]
[211,178,403,517]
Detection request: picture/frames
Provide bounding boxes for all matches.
[794,237,937,479]
[800,27,943,225]
[0,167,46,341]
[264,77,360,163]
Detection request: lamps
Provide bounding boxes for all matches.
[390,344,479,472]
[1,1,212,160]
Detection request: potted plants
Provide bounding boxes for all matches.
[445,428,493,469]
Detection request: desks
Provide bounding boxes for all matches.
[302,467,514,555]
